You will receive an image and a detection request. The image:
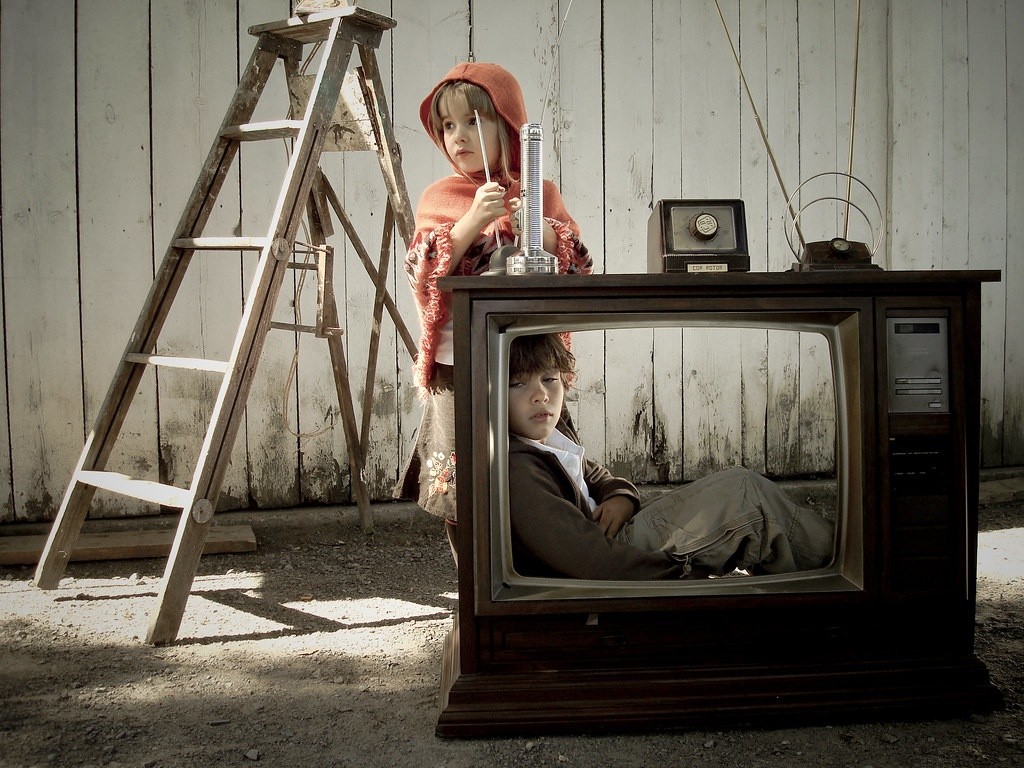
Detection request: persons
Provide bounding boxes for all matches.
[509,332,835,581]
[404,63,593,568]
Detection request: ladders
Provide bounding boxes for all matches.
[33,12,456,646]
